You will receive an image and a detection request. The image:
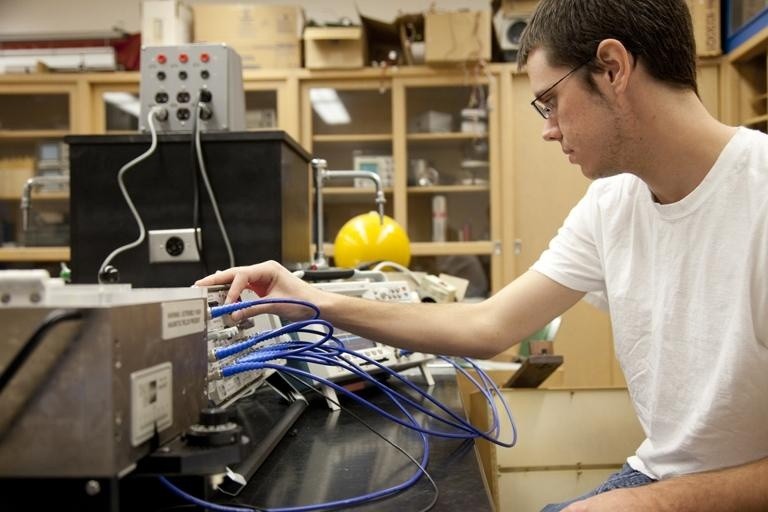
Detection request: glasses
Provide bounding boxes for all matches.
[530,58,594,119]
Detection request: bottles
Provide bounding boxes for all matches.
[431,195,447,242]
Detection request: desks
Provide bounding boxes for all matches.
[0,377,497,512]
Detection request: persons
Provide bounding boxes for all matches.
[180,0,767,511]
